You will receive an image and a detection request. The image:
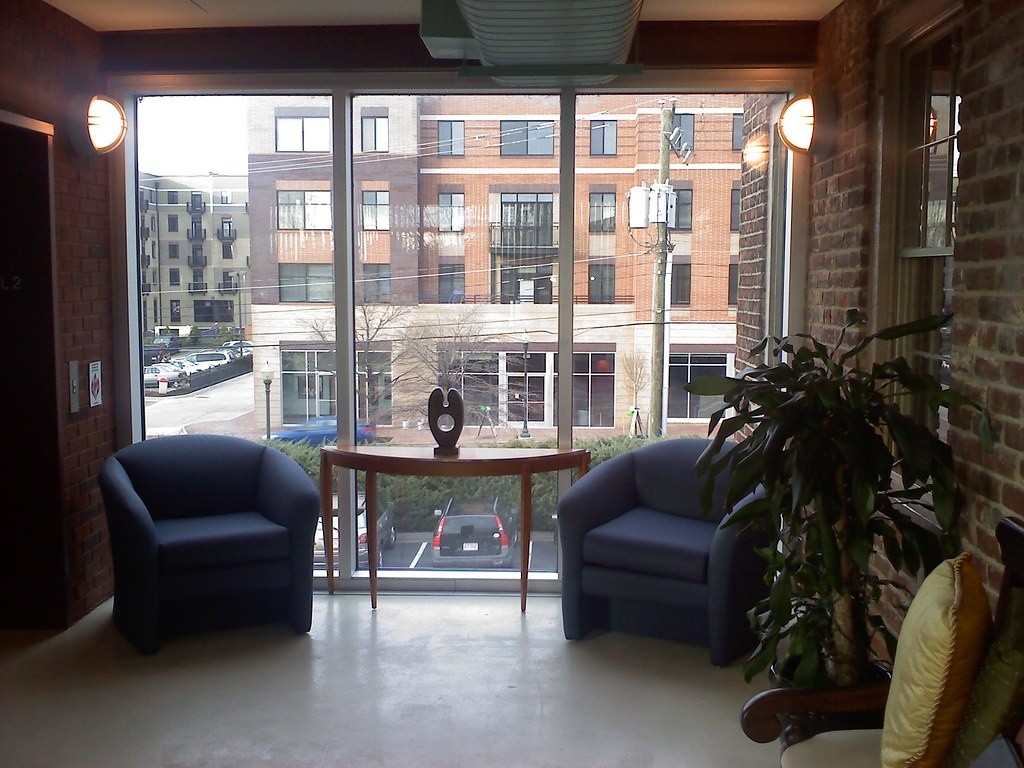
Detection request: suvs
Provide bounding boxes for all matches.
[222,341,253,352]
[434,497,518,570]
[313,492,397,568]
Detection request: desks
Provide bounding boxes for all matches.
[319,444,592,612]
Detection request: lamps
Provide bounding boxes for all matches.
[65,93,128,154]
[777,91,838,155]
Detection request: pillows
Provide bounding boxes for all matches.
[880,548,992,767]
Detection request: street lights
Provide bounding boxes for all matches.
[229,271,247,356]
[520,331,533,440]
[262,361,274,441]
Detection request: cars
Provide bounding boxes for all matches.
[185,351,235,365]
[260,416,376,446]
[202,347,251,357]
[153,335,183,354]
[143,366,184,387]
[144,343,171,366]
[164,358,214,377]
[147,363,187,380]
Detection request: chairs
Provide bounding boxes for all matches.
[740,515,1024,767]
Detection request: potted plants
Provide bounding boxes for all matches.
[684,308,994,748]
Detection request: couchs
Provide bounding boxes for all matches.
[559,439,782,665]
[100,434,322,653]
